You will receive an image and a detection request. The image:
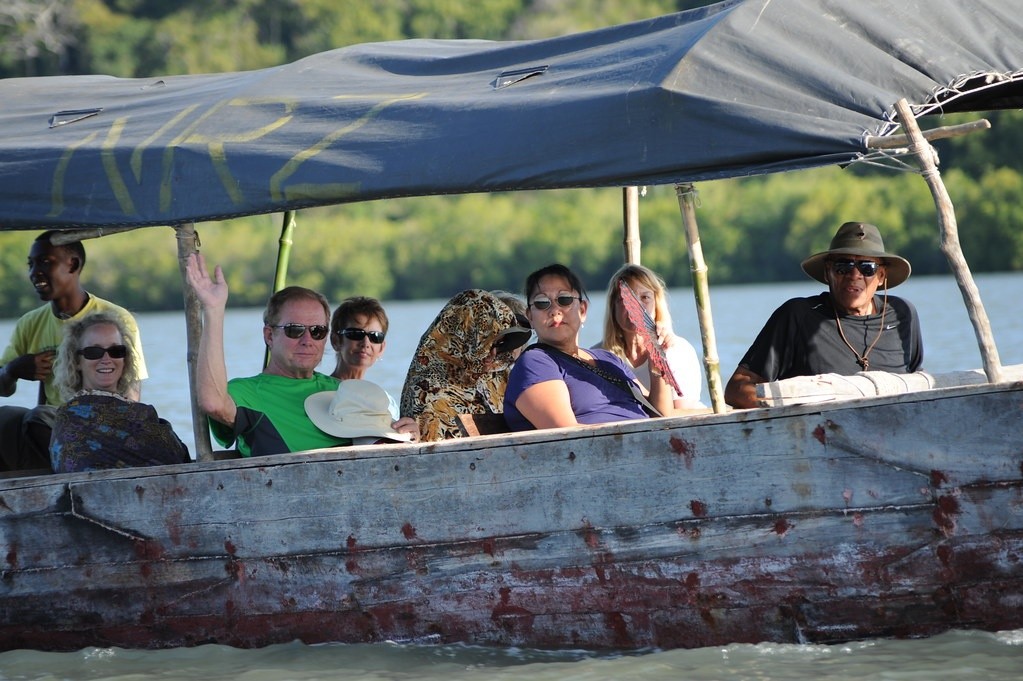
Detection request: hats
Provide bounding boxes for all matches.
[800,221,911,290]
[304,380,412,442]
[494,314,532,352]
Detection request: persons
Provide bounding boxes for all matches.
[723,222,924,410]
[590,263,703,412]
[503,262,677,433]
[326,297,389,383]
[50,310,192,473]
[185,251,422,457]
[0,230,149,471]
[400,289,538,442]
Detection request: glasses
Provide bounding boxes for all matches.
[75,344,127,361]
[490,340,506,351]
[829,258,884,277]
[271,322,328,340]
[529,292,582,310]
[336,327,385,344]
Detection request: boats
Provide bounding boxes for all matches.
[0,0,1023,661]
